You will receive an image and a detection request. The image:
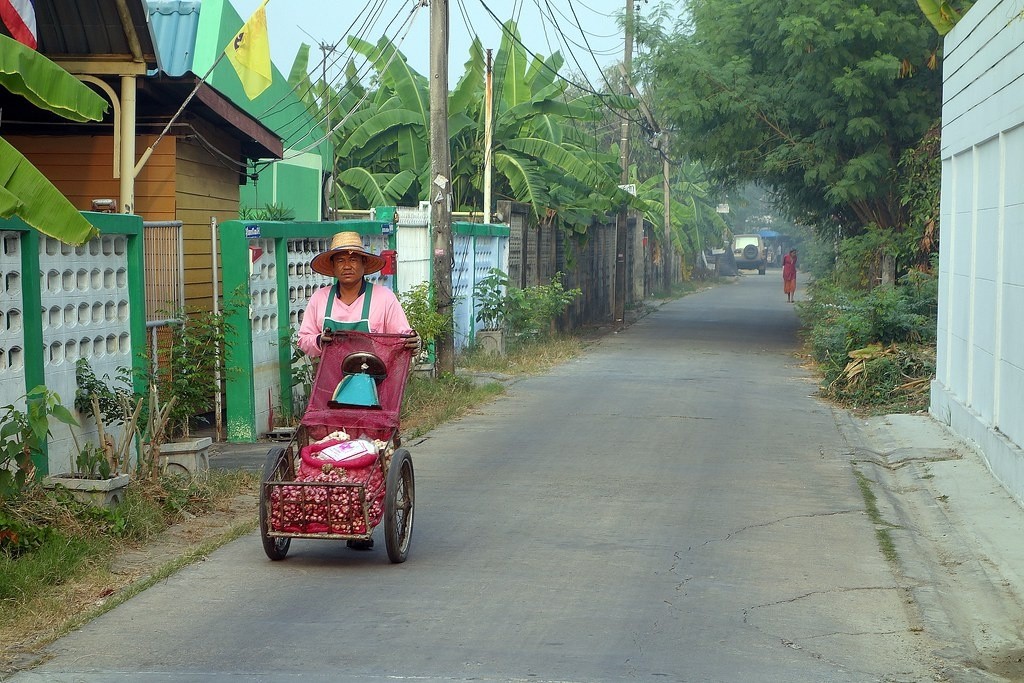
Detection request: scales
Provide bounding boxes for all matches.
[327,351,388,410]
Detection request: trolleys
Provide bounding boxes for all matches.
[258,328,418,564]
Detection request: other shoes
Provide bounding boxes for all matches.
[347,538,374,548]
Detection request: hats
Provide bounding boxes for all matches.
[310,232,386,277]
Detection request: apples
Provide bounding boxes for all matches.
[297,430,394,497]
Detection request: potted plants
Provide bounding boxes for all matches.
[475,266,531,355]
[146,281,248,484]
[411,284,456,378]
[42,448,131,508]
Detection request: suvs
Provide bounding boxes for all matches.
[729,234,767,275]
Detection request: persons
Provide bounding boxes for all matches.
[781,249,798,303]
[296,231,422,549]
